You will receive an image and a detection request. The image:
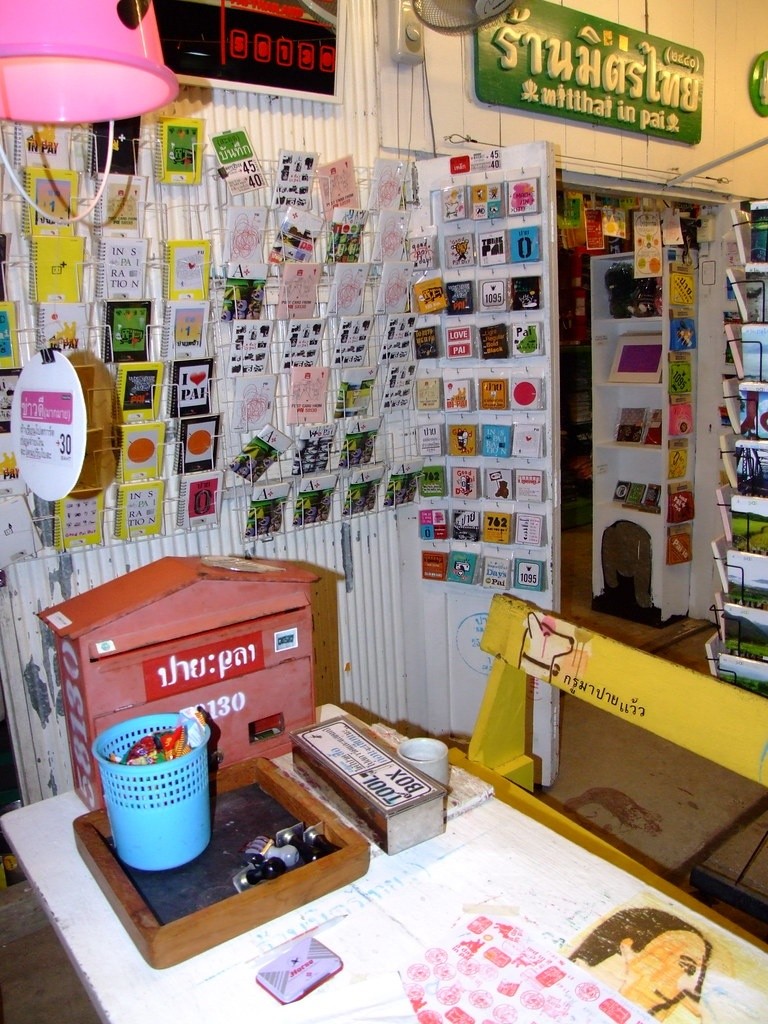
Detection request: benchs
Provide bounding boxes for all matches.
[441,595,768,956]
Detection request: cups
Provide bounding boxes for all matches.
[396,736,450,789]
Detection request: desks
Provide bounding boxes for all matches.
[0,703,768,1024]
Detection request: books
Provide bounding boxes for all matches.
[705,202,768,688]
[0,119,424,552]
[612,406,663,506]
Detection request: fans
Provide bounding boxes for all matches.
[387,0,522,68]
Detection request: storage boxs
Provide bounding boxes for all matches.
[72,755,370,970]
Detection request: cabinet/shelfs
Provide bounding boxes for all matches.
[588,247,689,631]
[702,195,768,705]
[560,341,592,529]
